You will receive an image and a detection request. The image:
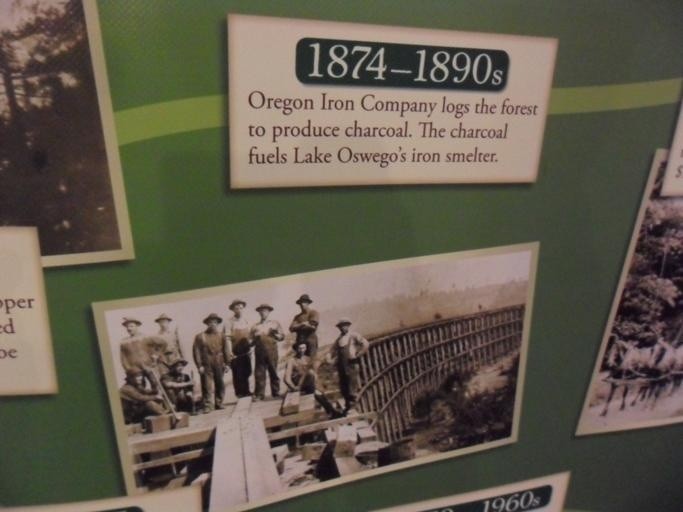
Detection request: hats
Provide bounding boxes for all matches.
[229,299,245,308]
[203,313,221,323]
[155,313,171,322]
[125,368,142,379]
[170,358,186,367]
[293,339,310,349]
[122,316,141,326]
[336,320,351,326]
[296,295,312,303]
[256,304,272,311]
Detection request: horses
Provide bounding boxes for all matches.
[597,339,652,418]
[646,336,683,412]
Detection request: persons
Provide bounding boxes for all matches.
[118,293,370,429]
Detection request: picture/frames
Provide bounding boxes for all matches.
[574,148,683,436]
[0,0,134,269]
[91,240,541,512]
